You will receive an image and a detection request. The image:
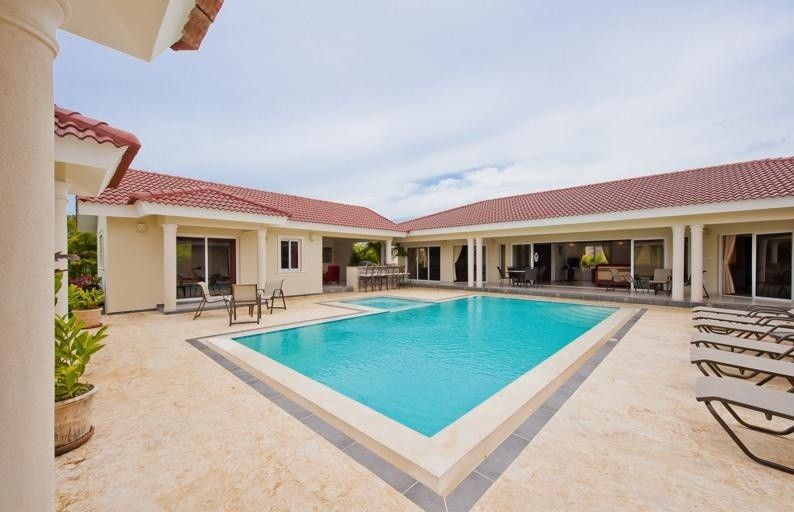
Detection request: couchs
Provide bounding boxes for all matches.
[593,264,630,288]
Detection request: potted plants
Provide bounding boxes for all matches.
[55,313,110,448]
[68,284,105,329]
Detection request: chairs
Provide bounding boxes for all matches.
[497,266,546,288]
[690,305,794,474]
[359,263,412,292]
[193,279,286,326]
[323,265,340,285]
[606,266,710,299]
